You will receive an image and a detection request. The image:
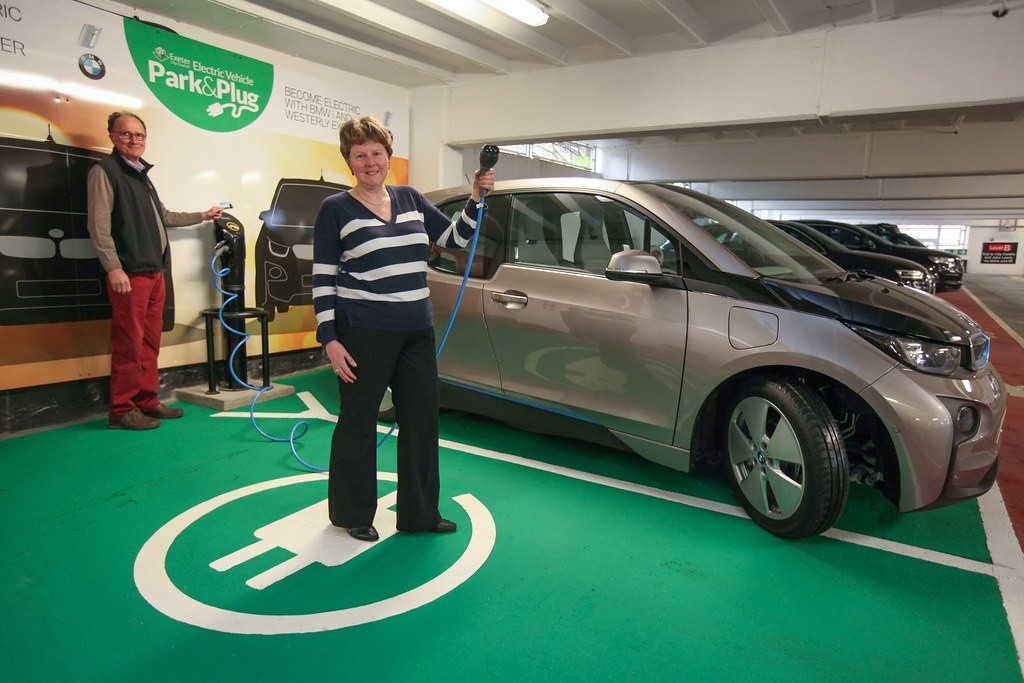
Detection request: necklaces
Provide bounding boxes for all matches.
[355,186,386,205]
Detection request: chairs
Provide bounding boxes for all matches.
[574,204,609,269]
[519,197,560,264]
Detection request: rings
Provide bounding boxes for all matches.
[335,370,339,372]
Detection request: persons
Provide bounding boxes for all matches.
[311,116,495,542]
[86,112,224,430]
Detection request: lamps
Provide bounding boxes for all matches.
[481,0,552,26]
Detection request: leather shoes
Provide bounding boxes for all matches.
[143,403,184,420]
[430,518,457,533]
[347,526,379,542]
[109,408,160,431]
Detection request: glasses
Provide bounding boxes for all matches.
[110,129,146,141]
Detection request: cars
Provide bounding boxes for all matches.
[650,219,967,305]
[375,178,1009,541]
